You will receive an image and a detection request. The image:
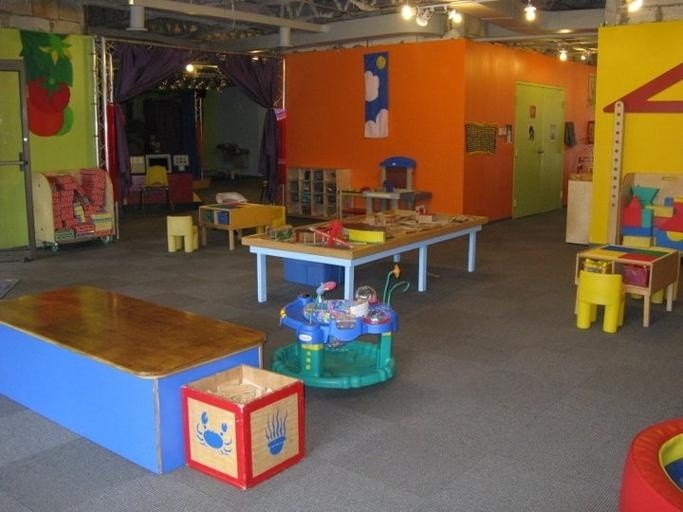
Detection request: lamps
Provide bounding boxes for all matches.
[398,2,470,28]
[623,0,643,14]
[523,1,536,21]
[558,47,570,63]
[158,62,227,95]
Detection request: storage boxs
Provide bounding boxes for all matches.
[181,363,305,491]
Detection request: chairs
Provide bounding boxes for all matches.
[142,185,175,215]
[573,269,626,334]
[165,215,198,256]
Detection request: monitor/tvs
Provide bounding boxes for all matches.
[145,153,173,174]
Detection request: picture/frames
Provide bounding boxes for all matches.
[588,120,595,145]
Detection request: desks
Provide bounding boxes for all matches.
[360,190,433,216]
[574,243,678,328]
[239,204,488,303]
[198,201,287,251]
[0,284,268,474]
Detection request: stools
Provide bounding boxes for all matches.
[619,417,683,512]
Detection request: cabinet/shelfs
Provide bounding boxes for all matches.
[565,177,592,246]
[284,168,350,219]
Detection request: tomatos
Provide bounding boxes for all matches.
[27,76,70,137]
